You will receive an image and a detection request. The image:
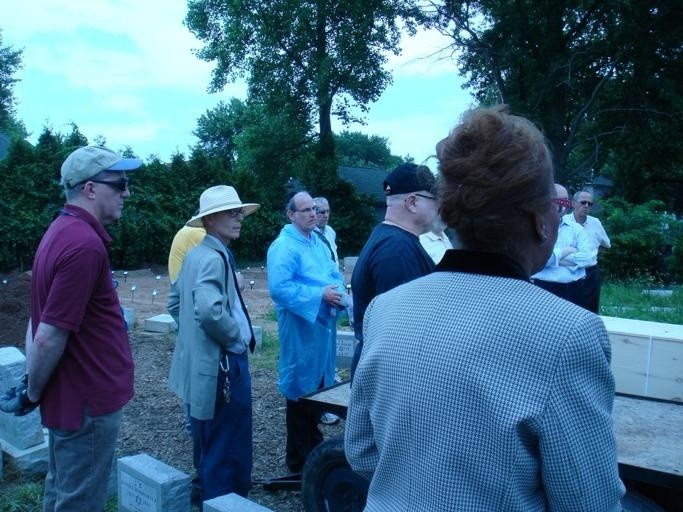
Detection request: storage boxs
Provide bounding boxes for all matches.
[596,314,683,403]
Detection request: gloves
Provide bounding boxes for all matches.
[0,374,39,416]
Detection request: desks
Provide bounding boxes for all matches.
[299,381,683,509]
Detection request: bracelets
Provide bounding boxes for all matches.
[22,374,28,384]
[19,388,39,409]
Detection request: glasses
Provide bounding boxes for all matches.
[230,207,245,217]
[94,177,130,191]
[551,197,571,216]
[579,199,593,206]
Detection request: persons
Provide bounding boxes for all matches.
[265,192,345,468]
[530,184,611,314]
[346,109,626,511]
[168,209,245,294]
[167,186,262,503]
[0,145,144,511]
[312,197,339,270]
[351,164,451,390]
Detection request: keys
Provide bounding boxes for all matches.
[221,371,232,405]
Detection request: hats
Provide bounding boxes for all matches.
[186,185,261,228]
[384,164,439,196]
[61,144,143,187]
